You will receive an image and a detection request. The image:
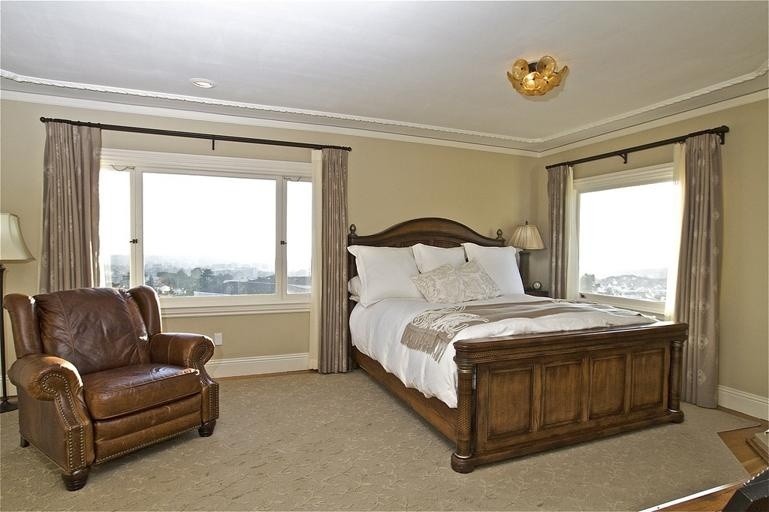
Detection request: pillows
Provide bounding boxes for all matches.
[347,242,525,308]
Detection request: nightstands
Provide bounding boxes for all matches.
[525,291,549,297]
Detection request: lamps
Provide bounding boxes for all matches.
[0,212,36,413]
[508,221,547,291]
[507,55,568,97]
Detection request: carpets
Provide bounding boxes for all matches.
[0,369,762,511]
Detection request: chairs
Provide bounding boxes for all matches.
[2,286,219,491]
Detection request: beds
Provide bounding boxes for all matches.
[348,218,689,474]
